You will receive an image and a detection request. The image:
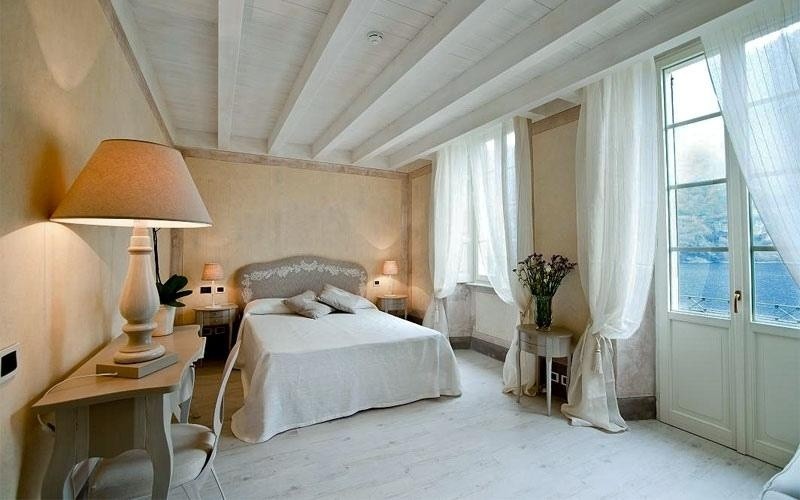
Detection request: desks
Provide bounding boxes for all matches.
[26,324,208,500]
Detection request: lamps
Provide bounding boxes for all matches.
[200,262,225,310]
[46,137,215,379]
[380,258,402,296]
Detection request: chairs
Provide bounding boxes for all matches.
[87,337,244,500]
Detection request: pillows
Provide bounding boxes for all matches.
[280,288,336,321]
[317,281,380,316]
[248,296,293,316]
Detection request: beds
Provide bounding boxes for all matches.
[228,254,464,446]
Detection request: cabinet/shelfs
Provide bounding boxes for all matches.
[513,323,572,417]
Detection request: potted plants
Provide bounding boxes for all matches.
[149,270,192,335]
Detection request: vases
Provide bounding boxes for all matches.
[531,295,553,329]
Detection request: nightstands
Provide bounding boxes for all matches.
[192,302,238,370]
[378,294,410,319]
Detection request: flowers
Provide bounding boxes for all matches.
[509,249,578,297]
